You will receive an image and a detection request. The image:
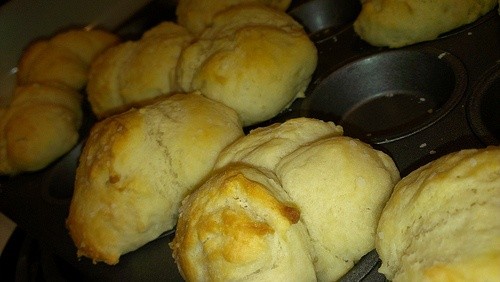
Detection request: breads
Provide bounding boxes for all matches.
[353,0,499,49]
[0,78,84,175]
[175,0,293,34]
[65,89,247,265]
[175,1,317,126]
[14,24,120,92]
[86,21,194,121]
[375,146,500,282]
[169,118,400,282]
